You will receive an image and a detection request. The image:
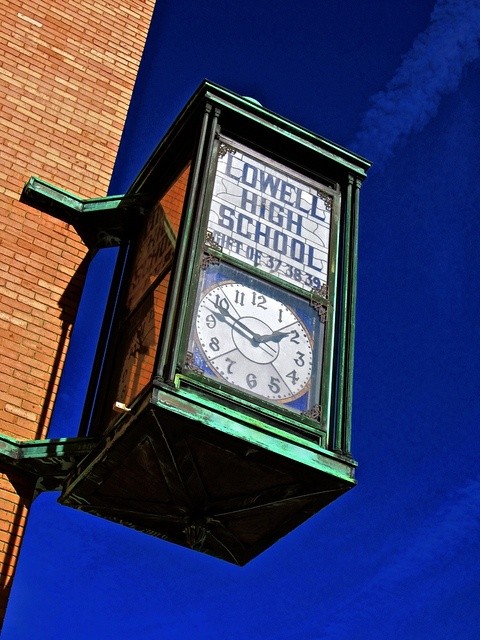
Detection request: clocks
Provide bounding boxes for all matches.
[107,261,176,428]
[176,244,334,432]
